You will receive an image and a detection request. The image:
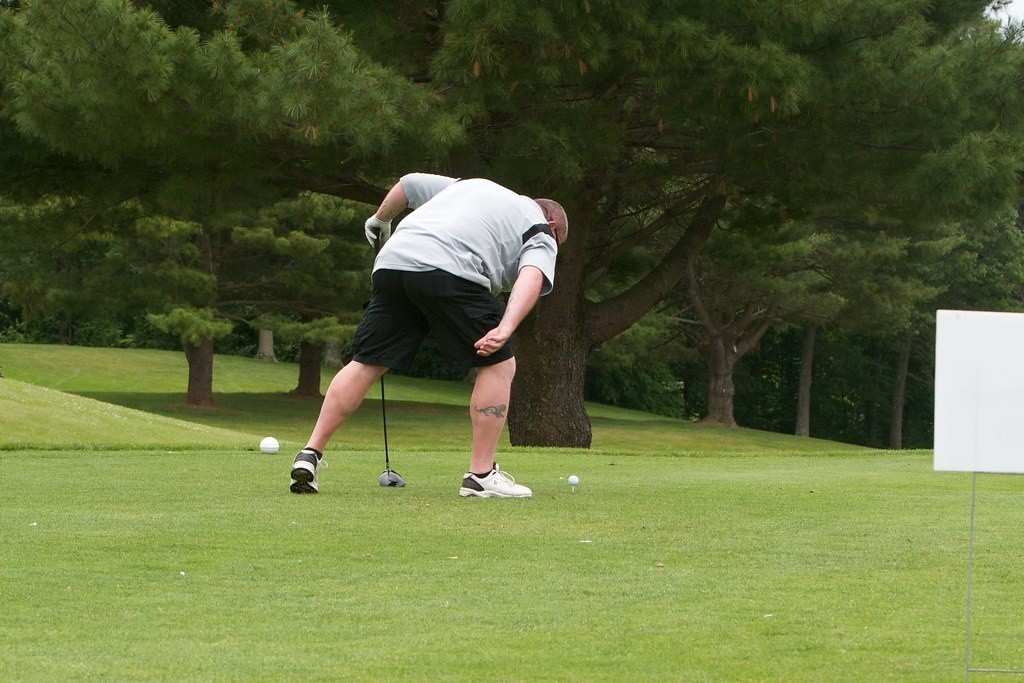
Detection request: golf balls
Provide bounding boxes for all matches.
[568,475,579,485]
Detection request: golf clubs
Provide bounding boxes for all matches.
[372,226,407,488]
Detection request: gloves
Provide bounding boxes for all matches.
[365,215,391,248]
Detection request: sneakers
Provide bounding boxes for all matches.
[290,450,329,493]
[460,462,533,497]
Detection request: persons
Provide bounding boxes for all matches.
[291,171,569,498]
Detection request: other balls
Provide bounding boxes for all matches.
[259,436,280,454]
